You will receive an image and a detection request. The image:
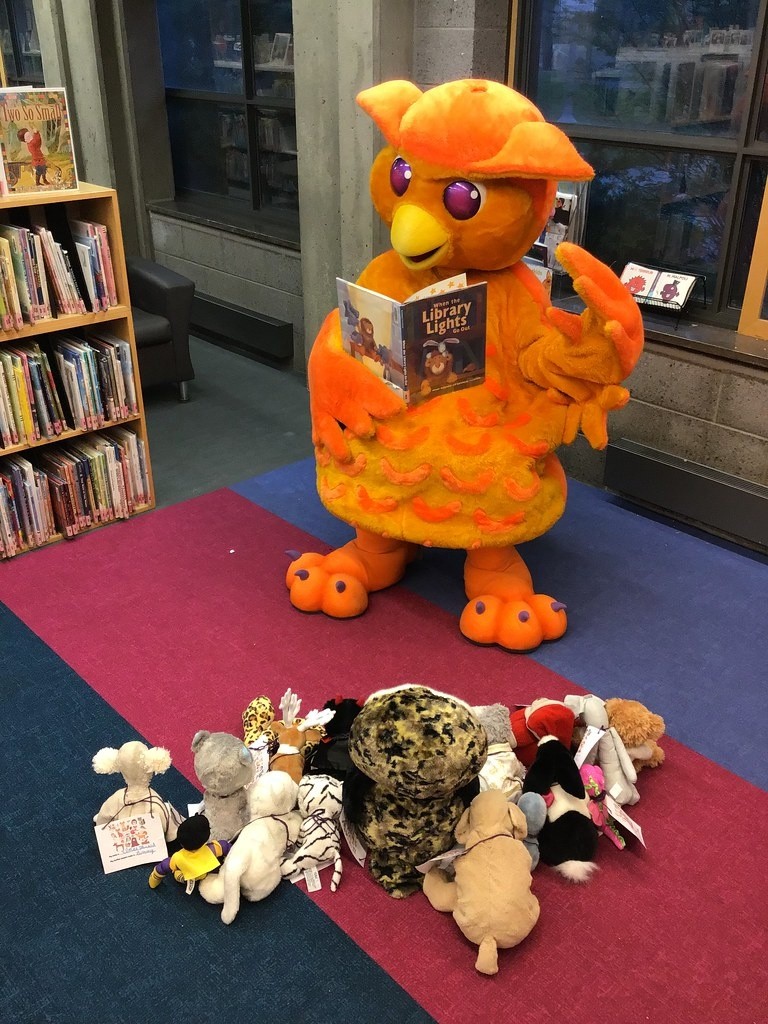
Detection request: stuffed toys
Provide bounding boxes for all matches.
[281,80,643,652]
[86,684,665,975]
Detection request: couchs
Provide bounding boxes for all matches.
[123,256,195,404]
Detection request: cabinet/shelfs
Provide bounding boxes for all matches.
[0,181,157,561]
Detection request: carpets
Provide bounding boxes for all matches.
[0,455,768,1024]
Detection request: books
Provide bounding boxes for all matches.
[619,261,697,309]
[521,190,578,298]
[0,218,120,331]
[334,272,487,405]
[1,424,151,562]
[1,336,139,451]
[0,83,77,197]
